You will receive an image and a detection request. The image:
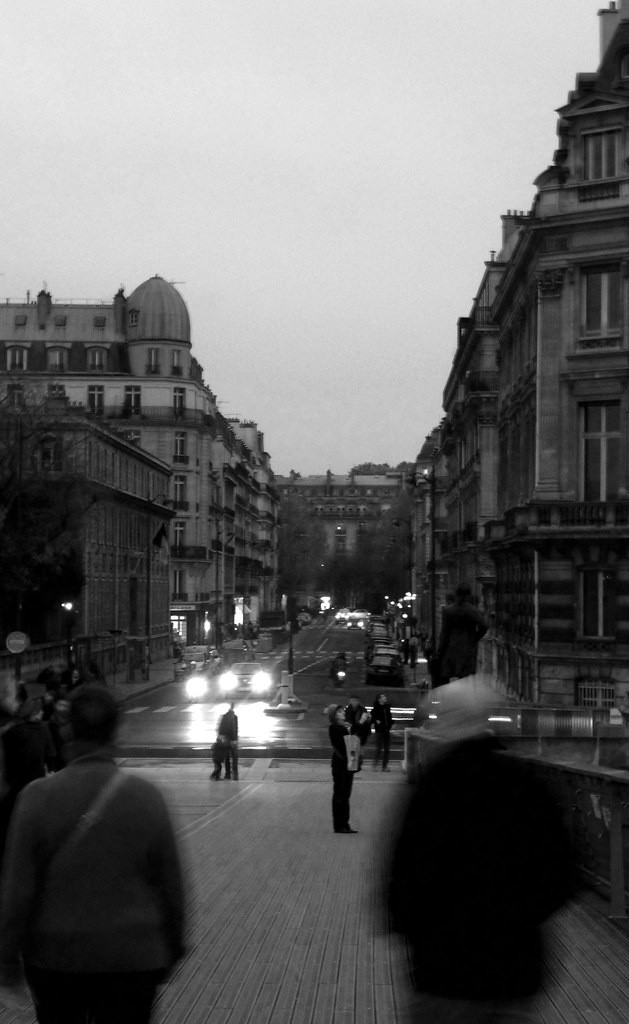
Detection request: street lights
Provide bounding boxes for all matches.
[215,518,233,650]
[404,463,437,660]
[146,490,174,664]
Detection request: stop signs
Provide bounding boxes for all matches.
[6,631,29,653]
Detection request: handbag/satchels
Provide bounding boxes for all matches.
[344,727,362,771]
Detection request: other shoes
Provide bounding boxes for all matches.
[381,768,390,772]
[334,829,359,833]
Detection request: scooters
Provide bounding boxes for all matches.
[328,661,352,688]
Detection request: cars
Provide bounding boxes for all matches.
[174,639,267,699]
[335,608,404,686]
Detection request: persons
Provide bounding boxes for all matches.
[140,651,153,682]
[127,648,137,681]
[0,659,191,1024]
[438,580,489,679]
[327,679,589,1024]
[329,660,340,681]
[209,708,240,780]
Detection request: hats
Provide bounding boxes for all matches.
[328,704,342,721]
